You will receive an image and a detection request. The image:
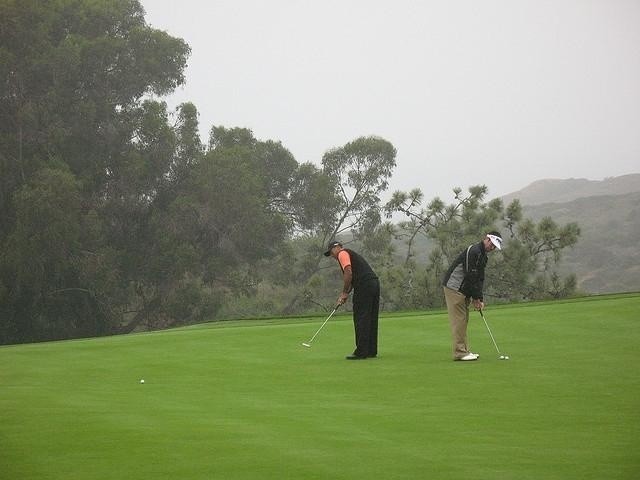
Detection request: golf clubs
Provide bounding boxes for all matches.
[302,304,340,348]
[478,306,502,354]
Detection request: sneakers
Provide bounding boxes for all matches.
[455,352,480,361]
[345,354,377,360]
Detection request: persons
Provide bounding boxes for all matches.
[320,240,382,361]
[440,231,504,362]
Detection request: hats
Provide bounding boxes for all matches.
[487,234,503,250]
[324,241,343,256]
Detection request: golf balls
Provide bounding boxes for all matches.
[499,356,504,359]
[140,379,144,384]
[505,356,508,360]
